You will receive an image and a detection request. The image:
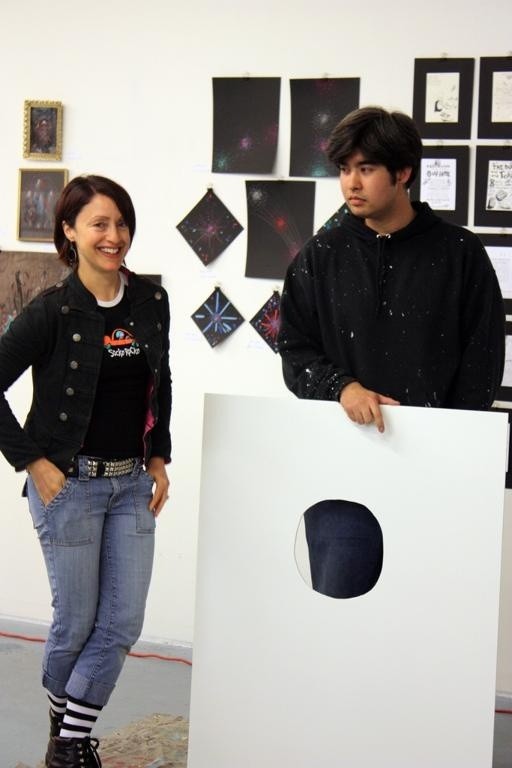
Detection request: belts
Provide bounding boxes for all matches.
[48,454,142,479]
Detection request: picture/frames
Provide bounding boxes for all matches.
[493,319,512,401]
[477,54,512,142]
[409,142,473,228]
[16,165,71,243]
[20,94,63,162]
[408,54,475,142]
[471,142,512,229]
[472,231,512,318]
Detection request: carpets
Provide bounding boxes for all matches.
[7,700,191,767]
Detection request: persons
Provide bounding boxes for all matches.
[0,173,173,767]
[20,176,60,231]
[34,115,55,152]
[276,99,508,601]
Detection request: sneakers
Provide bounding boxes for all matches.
[44,708,102,768]
